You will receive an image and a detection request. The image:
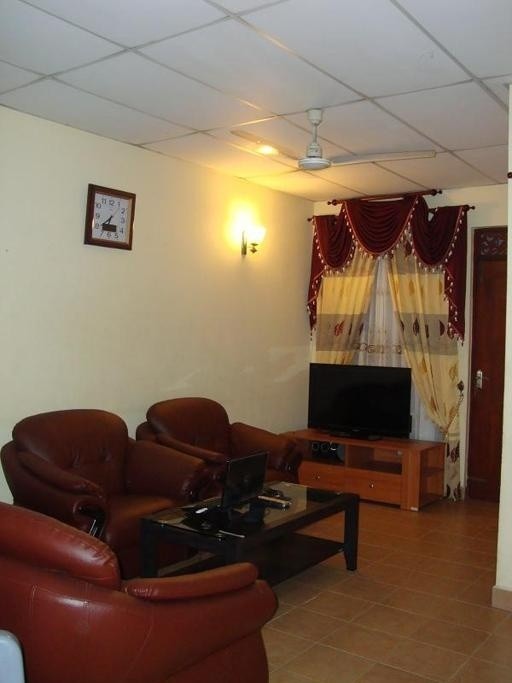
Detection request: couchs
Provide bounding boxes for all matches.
[1,409,224,579]
[135,397,297,500]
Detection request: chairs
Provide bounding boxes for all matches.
[3,500,276,683]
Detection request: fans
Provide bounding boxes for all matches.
[229,109,440,182]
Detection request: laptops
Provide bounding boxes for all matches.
[179,452,266,510]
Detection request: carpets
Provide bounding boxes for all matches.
[161,531,344,589]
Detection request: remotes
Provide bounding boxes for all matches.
[258,495,289,509]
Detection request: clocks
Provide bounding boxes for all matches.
[82,182,135,252]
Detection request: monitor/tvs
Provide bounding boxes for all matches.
[307,362,413,441]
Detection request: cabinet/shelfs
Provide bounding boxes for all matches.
[270,428,446,512]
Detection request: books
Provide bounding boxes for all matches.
[218,515,264,539]
